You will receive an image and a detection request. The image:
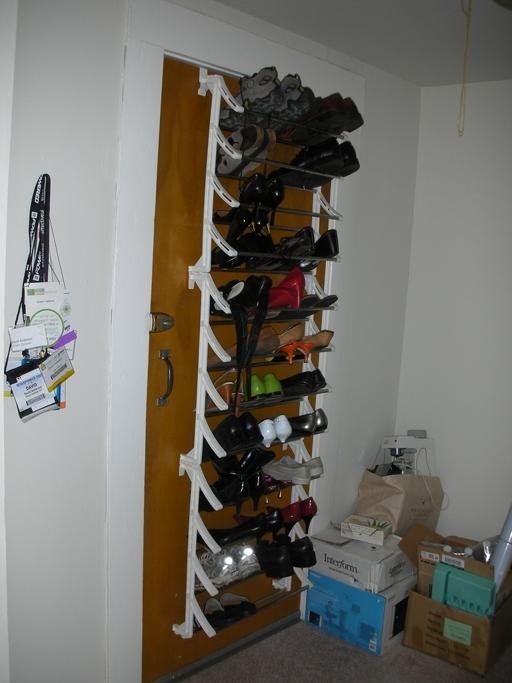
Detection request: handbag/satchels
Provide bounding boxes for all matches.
[352,467,444,538]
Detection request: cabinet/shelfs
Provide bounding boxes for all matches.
[171,66,337,643]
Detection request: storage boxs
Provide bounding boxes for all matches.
[304,515,512,677]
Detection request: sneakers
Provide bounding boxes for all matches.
[216,67,364,189]
